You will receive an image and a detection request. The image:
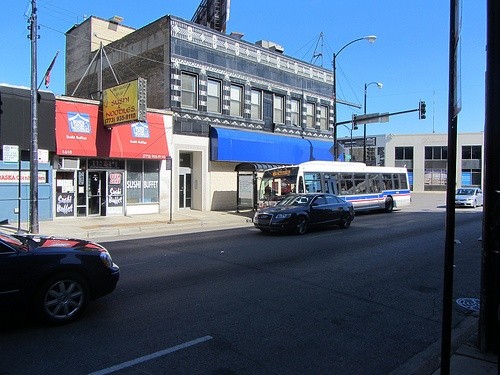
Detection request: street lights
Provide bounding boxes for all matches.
[333,35,378,163]
[363,81,384,166]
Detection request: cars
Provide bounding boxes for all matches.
[455,187,484,209]
[1,221,119,325]
[255,193,356,236]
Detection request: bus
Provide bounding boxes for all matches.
[256,160,412,213]
